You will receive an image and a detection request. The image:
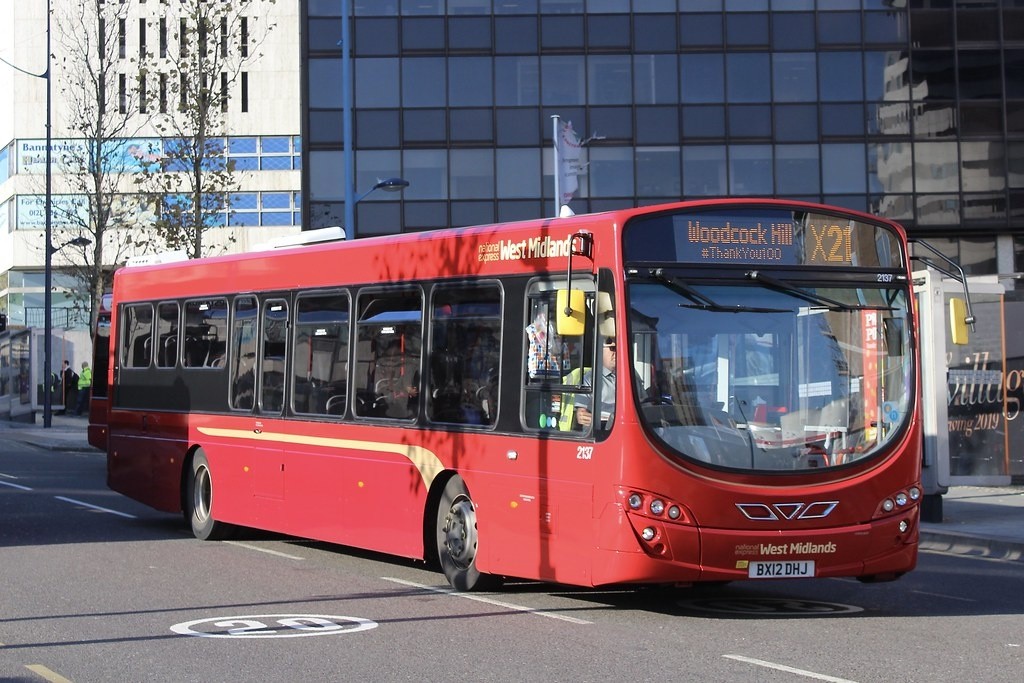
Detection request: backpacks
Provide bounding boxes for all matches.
[54,374,58,388]
[72,372,79,384]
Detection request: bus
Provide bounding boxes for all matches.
[87,197,978,593]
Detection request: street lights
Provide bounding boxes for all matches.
[343,175,411,241]
[43,236,93,428]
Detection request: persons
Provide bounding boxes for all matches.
[44,360,91,417]
[215,316,672,435]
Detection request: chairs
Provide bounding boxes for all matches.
[125,324,789,437]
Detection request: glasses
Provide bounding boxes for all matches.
[610,346,616,351]
